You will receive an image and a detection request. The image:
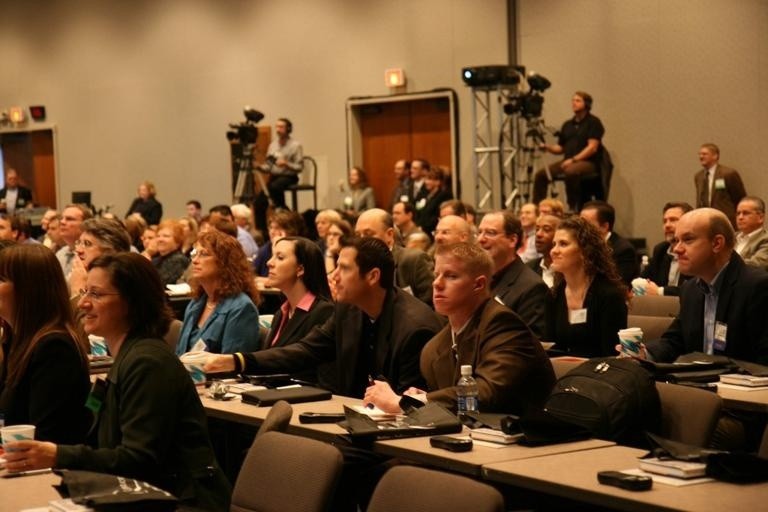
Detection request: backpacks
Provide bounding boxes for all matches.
[543,356,662,448]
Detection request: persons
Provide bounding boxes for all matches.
[416,169,448,244]
[175,230,260,376]
[41,208,58,248]
[202,234,444,396]
[0,251,232,512]
[0,213,41,244]
[230,205,254,233]
[542,215,630,359]
[0,244,91,448]
[123,213,146,253]
[124,181,163,226]
[580,202,640,292]
[325,219,352,279]
[55,205,93,278]
[47,215,67,253]
[337,165,375,226]
[251,213,299,278]
[355,207,437,311]
[533,213,563,288]
[185,200,203,224]
[515,203,544,265]
[65,218,131,358]
[641,201,695,296]
[475,212,551,341]
[198,215,238,238]
[464,204,478,237]
[539,199,564,216]
[410,158,431,206]
[178,216,198,258]
[392,202,430,252]
[614,207,768,360]
[140,227,156,249]
[209,204,258,258]
[0,168,33,219]
[432,214,470,247]
[693,143,747,232]
[387,159,413,215]
[148,220,193,292]
[532,91,606,205]
[262,236,336,350]
[254,118,304,229]
[437,199,467,221]
[266,207,289,229]
[314,209,341,253]
[361,241,557,417]
[733,195,768,269]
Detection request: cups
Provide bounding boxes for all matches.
[179,352,208,395]
[616,328,644,358]
[0,427,36,475]
[88,334,109,357]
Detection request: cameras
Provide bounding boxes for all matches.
[504,72,552,118]
[226,106,266,145]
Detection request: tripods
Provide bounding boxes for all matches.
[505,123,560,215]
[225,146,275,211]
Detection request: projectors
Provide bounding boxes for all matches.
[462,65,525,88]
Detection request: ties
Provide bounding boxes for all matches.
[702,173,710,208]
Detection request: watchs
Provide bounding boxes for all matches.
[572,156,576,162]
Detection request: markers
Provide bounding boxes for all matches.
[367,374,374,386]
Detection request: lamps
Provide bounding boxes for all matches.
[9,107,27,124]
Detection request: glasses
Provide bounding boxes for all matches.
[327,232,341,236]
[78,290,118,301]
[431,230,459,235]
[192,250,215,260]
[73,238,96,249]
[478,229,511,236]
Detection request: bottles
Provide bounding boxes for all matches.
[640,256,650,272]
[456,365,479,429]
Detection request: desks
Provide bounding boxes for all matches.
[0,464,94,512]
[482,443,768,512]
[200,380,464,445]
[377,426,620,483]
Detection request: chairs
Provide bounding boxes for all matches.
[365,463,504,512]
[228,432,343,512]
[286,157,318,211]
[546,359,578,381]
[258,401,293,437]
[625,293,682,348]
[656,381,723,448]
